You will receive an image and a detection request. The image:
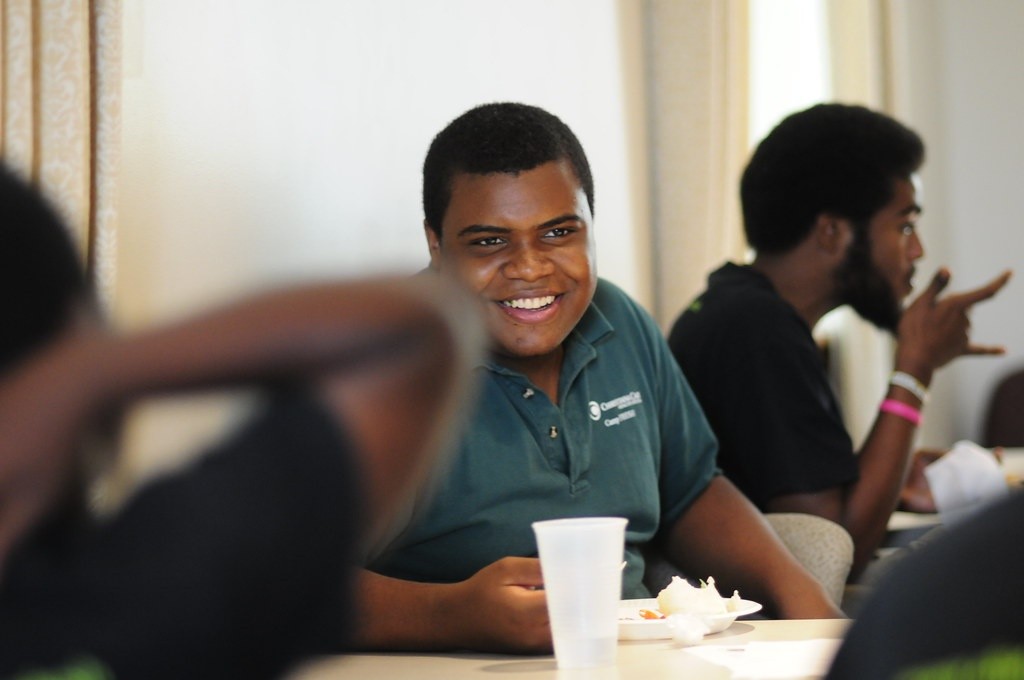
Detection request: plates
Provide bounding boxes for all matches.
[616,598,763,640]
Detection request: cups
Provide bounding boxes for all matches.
[530,517,629,674]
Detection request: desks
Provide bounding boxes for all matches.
[288,618,854,680]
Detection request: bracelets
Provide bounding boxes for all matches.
[880,399,920,424]
[888,370,931,403]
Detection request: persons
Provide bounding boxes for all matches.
[354,103,850,653]
[0,160,463,680]
[667,104,1012,586]
